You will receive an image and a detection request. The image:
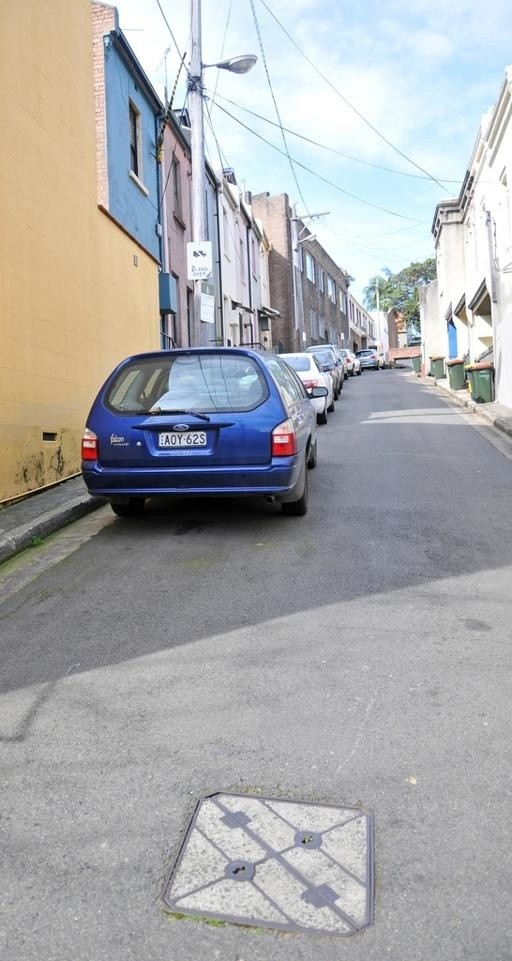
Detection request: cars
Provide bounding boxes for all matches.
[276,353,335,426]
[80,346,317,515]
[307,344,348,402]
[339,349,361,377]
[355,349,385,372]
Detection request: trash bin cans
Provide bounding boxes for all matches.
[447,360,495,403]
[429,355,443,378]
[412,355,421,373]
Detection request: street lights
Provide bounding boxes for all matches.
[292,234,322,352]
[188,53,258,349]
